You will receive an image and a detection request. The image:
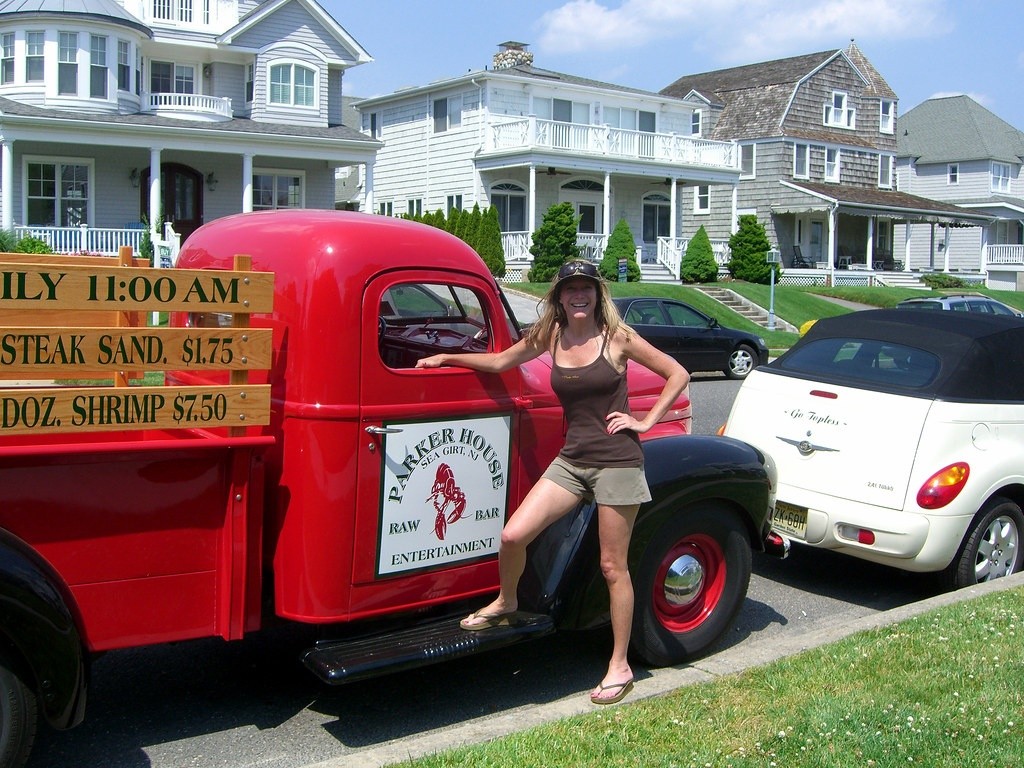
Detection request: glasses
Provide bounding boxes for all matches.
[558,263,597,276]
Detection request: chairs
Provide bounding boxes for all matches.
[878,248,905,272]
[641,314,656,325]
[793,245,816,268]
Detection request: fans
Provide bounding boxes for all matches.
[649,178,686,186]
[536,167,572,176]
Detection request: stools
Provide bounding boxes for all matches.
[838,256,852,269]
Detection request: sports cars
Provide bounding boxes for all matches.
[714,305,1024,599]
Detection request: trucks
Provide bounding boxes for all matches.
[1,209,791,768]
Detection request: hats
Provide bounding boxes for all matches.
[552,259,601,287]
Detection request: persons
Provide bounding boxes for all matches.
[415,260,691,704]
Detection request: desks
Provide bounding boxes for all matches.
[873,261,884,271]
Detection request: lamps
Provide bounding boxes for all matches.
[130,168,142,188]
[206,171,218,191]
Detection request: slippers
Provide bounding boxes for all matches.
[460,607,519,630]
[591,678,634,704]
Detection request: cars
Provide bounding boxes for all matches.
[378,279,527,343]
[879,292,1024,369]
[609,295,769,382]
